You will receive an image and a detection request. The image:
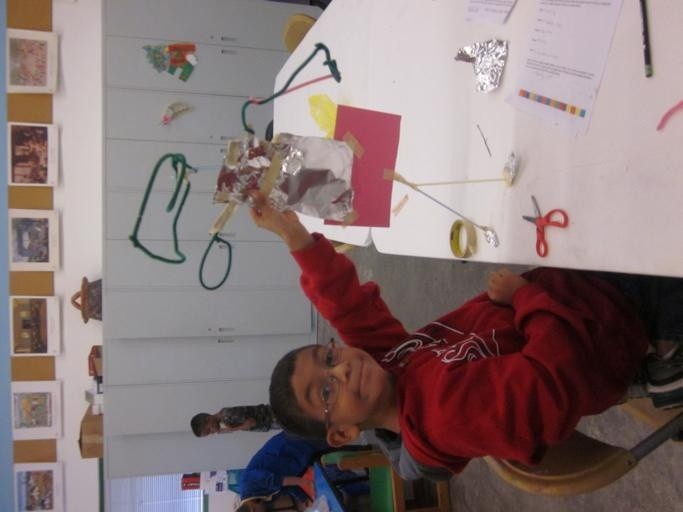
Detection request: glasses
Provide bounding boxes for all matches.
[316,336,345,434]
